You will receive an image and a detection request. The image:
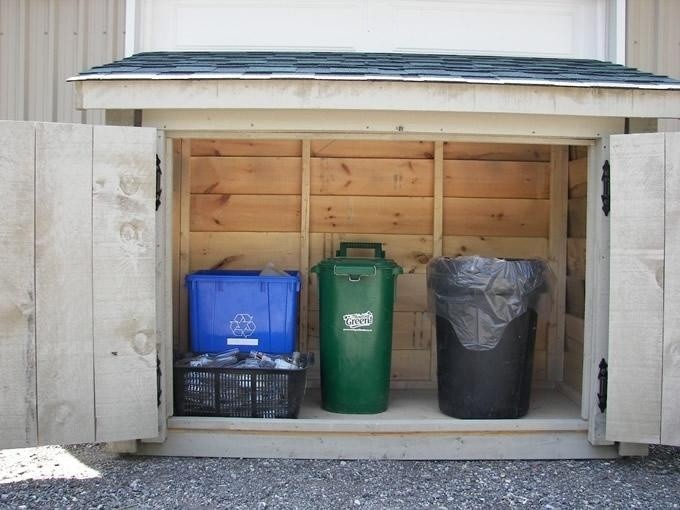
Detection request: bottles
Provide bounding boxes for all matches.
[184,347,305,370]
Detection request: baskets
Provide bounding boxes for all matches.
[173,352,309,419]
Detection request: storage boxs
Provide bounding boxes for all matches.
[183,267,303,355]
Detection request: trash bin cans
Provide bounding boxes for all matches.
[426,255,548,420]
[309,256,404,416]
